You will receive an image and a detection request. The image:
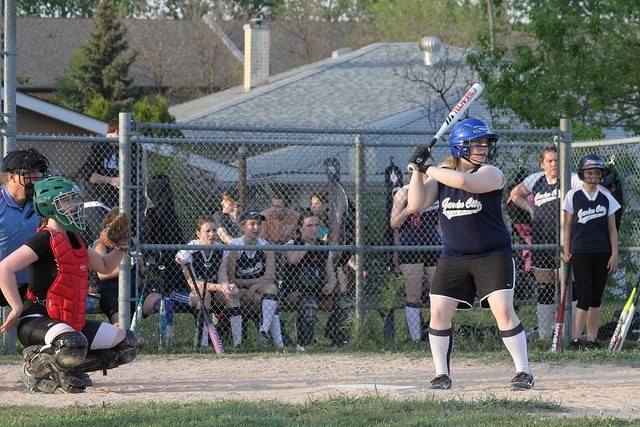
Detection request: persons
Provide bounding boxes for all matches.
[259,189,303,287]
[561,153,622,351]
[0,148,52,307]
[140,173,190,292]
[278,209,352,351]
[406,115,534,391]
[222,210,292,353]
[211,187,248,245]
[163,215,246,353]
[131,118,148,232]
[309,190,368,298]
[509,145,587,343]
[75,117,120,239]
[91,206,175,346]
[0,176,139,394]
[503,167,536,272]
[389,156,443,350]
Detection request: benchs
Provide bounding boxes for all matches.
[87,300,403,349]
[404,298,537,346]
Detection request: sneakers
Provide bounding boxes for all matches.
[431,374,452,390]
[49,371,91,395]
[259,327,271,344]
[585,337,601,351]
[19,363,42,393]
[569,338,582,348]
[510,372,534,390]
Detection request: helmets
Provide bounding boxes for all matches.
[578,155,608,184]
[3,148,53,201]
[449,118,500,166]
[32,176,86,235]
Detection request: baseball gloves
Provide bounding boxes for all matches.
[99,205,131,250]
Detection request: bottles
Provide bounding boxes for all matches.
[230,283,240,308]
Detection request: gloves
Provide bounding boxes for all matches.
[409,152,433,175]
[414,145,434,160]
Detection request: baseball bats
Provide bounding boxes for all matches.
[130,261,153,340]
[183,263,223,357]
[607,274,640,351]
[194,263,212,355]
[160,265,168,351]
[612,287,640,353]
[551,261,572,353]
[407,83,483,172]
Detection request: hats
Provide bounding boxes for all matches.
[240,210,267,225]
